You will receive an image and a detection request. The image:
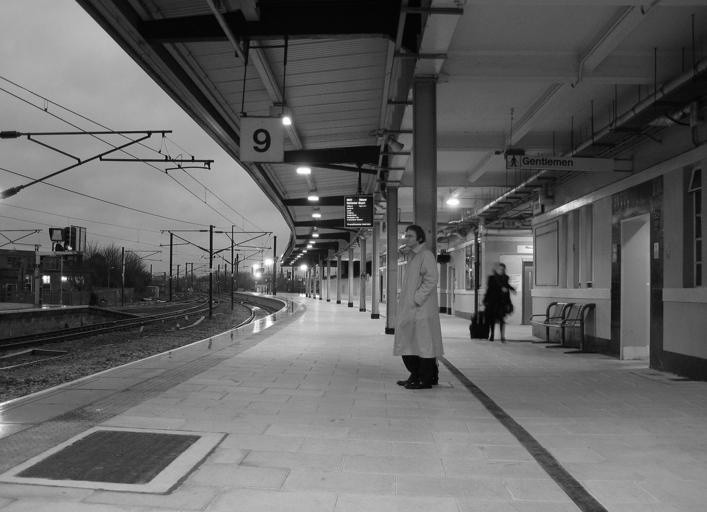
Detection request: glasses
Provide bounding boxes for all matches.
[405,235,412,237]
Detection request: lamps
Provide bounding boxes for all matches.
[282,111,322,265]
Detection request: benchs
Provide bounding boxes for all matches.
[528,302,597,353]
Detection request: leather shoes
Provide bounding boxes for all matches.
[489,337,505,341]
[396,372,438,389]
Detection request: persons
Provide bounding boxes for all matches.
[392,224,445,391]
[483,262,517,343]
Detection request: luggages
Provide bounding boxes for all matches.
[469,311,489,339]
